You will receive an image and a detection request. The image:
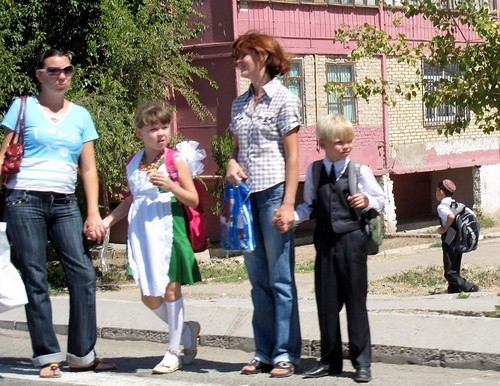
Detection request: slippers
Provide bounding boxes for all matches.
[70,358,116,372]
[39,362,62,378]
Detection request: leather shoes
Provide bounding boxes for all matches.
[355,367,372,382]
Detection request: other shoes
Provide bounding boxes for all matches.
[182,321,201,364]
[302,364,342,377]
[153,349,182,373]
[468,285,479,292]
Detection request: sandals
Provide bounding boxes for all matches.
[242,359,271,374]
[271,362,300,377]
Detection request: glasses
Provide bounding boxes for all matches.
[37,66,75,78]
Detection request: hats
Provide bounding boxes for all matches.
[442,179,457,192]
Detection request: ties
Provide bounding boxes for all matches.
[329,164,337,182]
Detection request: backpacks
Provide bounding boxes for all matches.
[126,149,209,253]
[312,159,384,254]
[441,201,479,255]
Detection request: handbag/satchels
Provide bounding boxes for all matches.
[1,96,27,183]
[221,180,257,254]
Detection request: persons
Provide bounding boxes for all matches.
[431,175,483,295]
[82,100,206,375]
[223,31,306,379]
[0,47,116,381]
[273,113,387,383]
